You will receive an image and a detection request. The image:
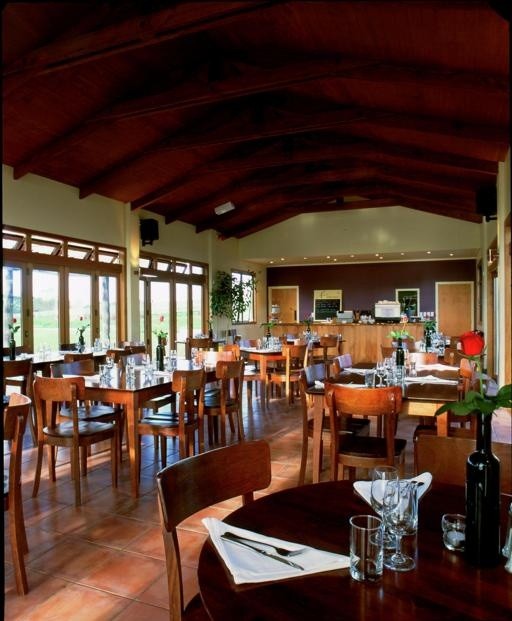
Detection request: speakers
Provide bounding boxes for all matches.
[476,182,496,215]
[140,219,159,240]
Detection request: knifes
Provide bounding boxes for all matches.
[219,535,303,572]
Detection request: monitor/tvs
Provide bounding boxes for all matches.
[337,311,355,319]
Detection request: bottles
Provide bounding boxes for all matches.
[501,497,512,573]
[313,330,342,343]
[254,335,283,353]
[359,312,409,324]
[461,410,496,548]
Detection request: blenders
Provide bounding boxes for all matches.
[269,305,282,323]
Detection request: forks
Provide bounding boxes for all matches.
[224,532,310,556]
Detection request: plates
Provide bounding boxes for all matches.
[286,338,300,342]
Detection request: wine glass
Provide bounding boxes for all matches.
[366,349,415,399]
[371,466,419,572]
[417,333,451,356]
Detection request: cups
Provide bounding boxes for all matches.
[442,515,465,552]
[349,515,385,583]
[94,336,205,383]
